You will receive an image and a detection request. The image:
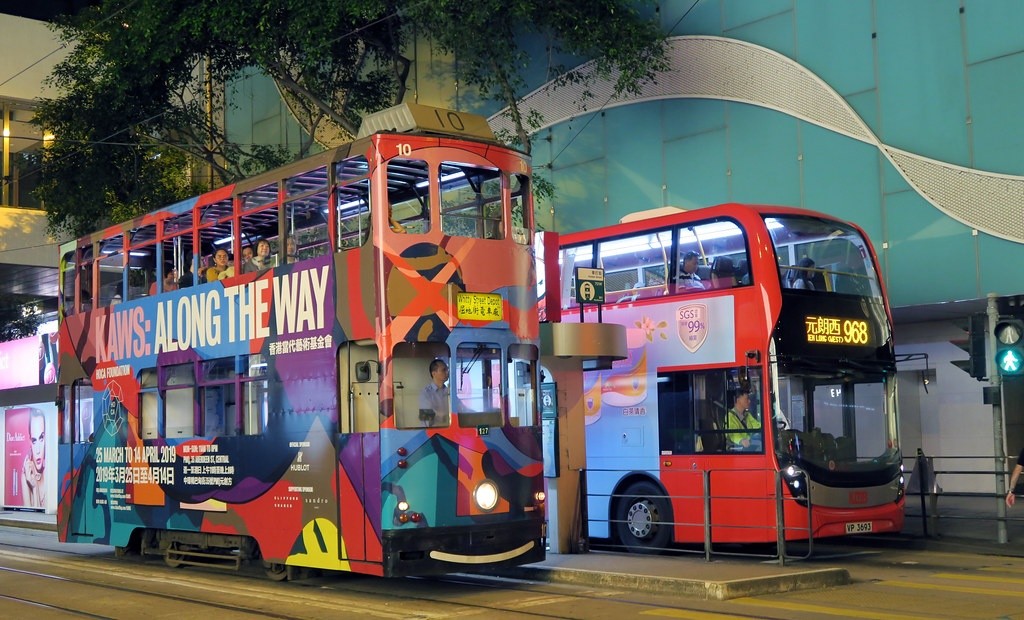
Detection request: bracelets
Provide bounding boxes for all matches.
[1008,490,1014,492]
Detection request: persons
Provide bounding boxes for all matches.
[491,206,513,239]
[723,389,762,448]
[419,359,468,427]
[1005,448,1024,508]
[365,200,407,241]
[110,281,123,305]
[679,251,705,289]
[783,257,815,290]
[206,236,297,284]
[148,249,208,296]
[21,408,47,508]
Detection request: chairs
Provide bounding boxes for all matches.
[697,257,748,289]
[781,265,796,288]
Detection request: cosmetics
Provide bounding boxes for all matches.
[12,469,18,496]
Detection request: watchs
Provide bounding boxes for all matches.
[35,473,41,481]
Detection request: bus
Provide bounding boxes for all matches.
[455,203,931,549]
[57,100,548,585]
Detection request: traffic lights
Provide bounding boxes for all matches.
[996,320,1023,376]
[949,313,987,380]
